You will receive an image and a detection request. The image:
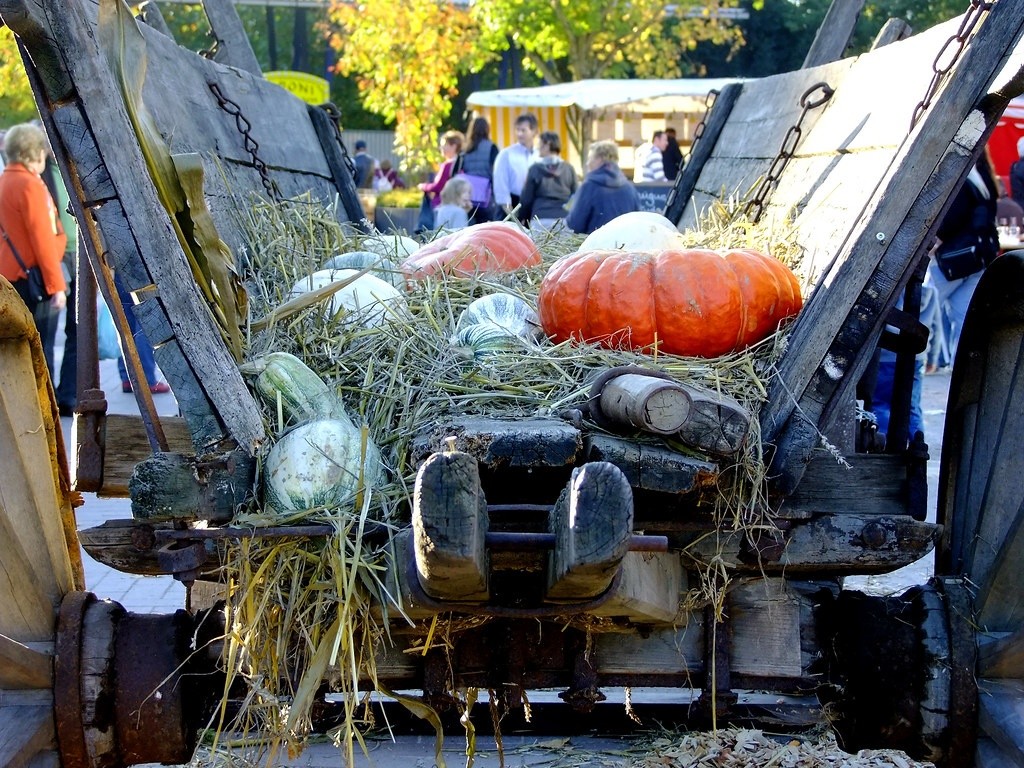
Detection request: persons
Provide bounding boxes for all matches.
[994,137,1024,226]
[118,320,171,394]
[418,113,578,236]
[633,128,686,183]
[921,144,1000,376]
[351,140,406,192]
[0,124,68,387]
[566,139,642,236]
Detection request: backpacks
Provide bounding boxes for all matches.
[377,169,395,192]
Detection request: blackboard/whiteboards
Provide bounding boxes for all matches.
[630,179,677,218]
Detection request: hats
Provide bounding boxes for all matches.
[356,141,365,147]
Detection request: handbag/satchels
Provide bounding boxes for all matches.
[936,242,987,281]
[413,192,434,234]
[454,173,491,208]
[28,265,53,302]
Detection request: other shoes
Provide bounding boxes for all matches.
[149,382,170,393]
[925,364,938,375]
[123,381,133,393]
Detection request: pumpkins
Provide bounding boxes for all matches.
[286,220,544,365]
[538,212,802,358]
[234,352,386,517]
